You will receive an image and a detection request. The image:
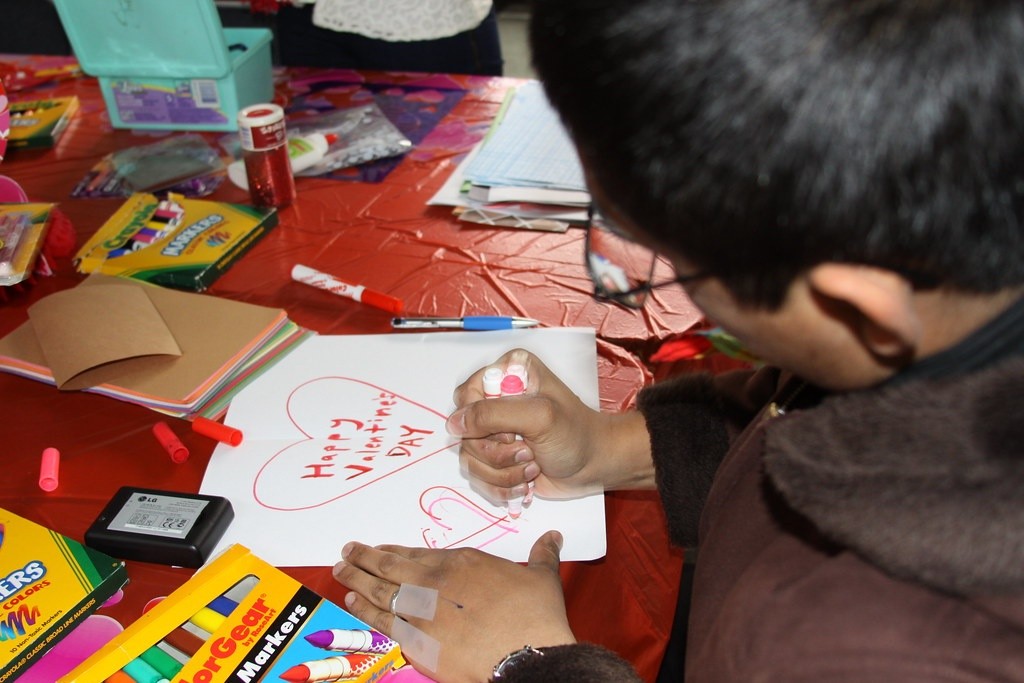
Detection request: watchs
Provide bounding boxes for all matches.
[492,645,554,681]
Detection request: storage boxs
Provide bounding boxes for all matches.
[51,0,274,132]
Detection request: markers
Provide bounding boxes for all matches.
[480,363,538,518]
[289,261,402,315]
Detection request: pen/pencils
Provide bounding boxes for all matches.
[389,313,546,331]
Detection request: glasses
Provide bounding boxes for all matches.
[582,201,744,311]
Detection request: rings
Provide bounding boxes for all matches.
[390,589,400,614]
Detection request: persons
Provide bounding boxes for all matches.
[291,0,505,76]
[333,0,1024,683]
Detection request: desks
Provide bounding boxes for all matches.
[0,53,746,682]
